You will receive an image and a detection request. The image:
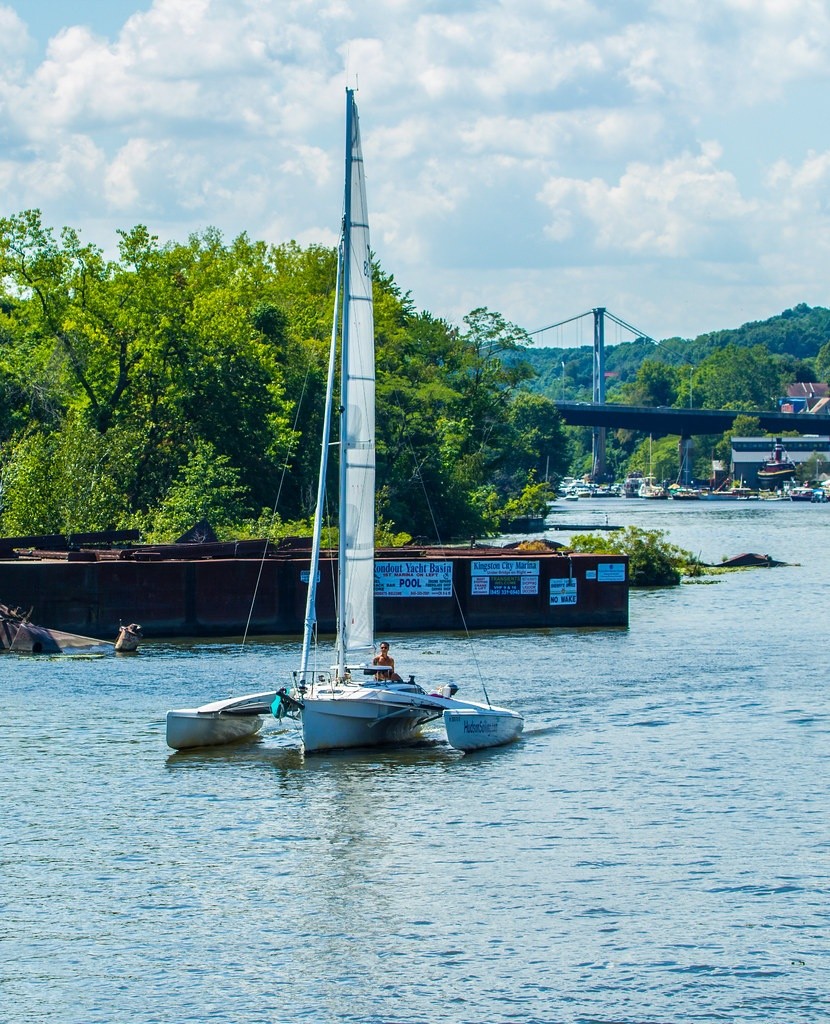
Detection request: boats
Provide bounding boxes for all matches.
[558,430,830,504]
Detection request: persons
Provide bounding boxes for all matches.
[372,642,395,682]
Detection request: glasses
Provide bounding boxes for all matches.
[380,646,388,649]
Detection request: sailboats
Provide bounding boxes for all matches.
[165,74,525,760]
